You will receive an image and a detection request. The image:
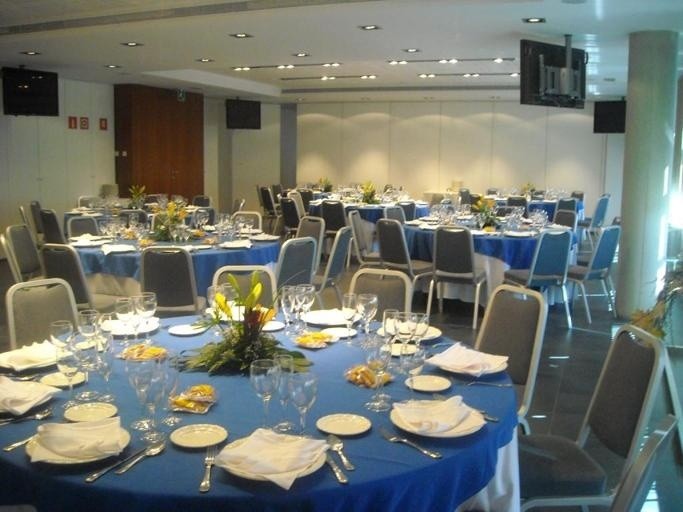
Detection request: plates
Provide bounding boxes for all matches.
[0,342,87,414]
[23,436,131,466]
[407,216,567,237]
[382,323,511,439]
[310,199,426,208]
[214,436,326,482]
[168,424,229,450]
[67,233,281,253]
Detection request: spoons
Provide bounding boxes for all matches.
[326,432,356,473]
[0,409,49,425]
[114,440,167,475]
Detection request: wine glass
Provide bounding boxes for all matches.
[91,194,253,242]
[50,283,432,443]
[425,185,567,231]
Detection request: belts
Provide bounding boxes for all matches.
[425,185,567,231]
[91,194,253,242]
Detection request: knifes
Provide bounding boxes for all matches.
[84,447,147,483]
[324,455,348,484]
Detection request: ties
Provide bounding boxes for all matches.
[3,68,59,117]
[226,98,264,131]
[593,100,628,133]
[518,37,588,109]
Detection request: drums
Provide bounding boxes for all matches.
[324,455,348,484]
[84,447,147,483]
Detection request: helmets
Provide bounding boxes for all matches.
[23,436,131,466]
[67,233,281,253]
[382,323,511,439]
[214,436,326,482]
[407,216,567,237]
[310,199,426,208]
[0,342,87,414]
[168,424,229,450]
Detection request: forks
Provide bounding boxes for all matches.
[377,426,445,460]
[198,442,216,493]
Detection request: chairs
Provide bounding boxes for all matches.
[0,183,683,512]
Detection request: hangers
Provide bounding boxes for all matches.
[0,409,49,425]
[326,432,356,473]
[114,440,167,475]
